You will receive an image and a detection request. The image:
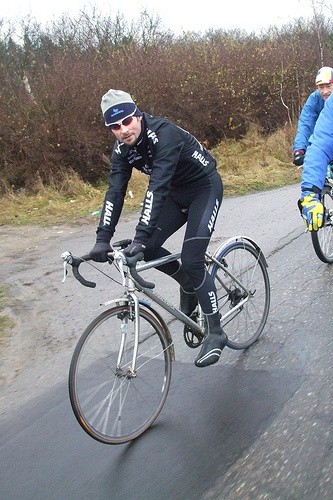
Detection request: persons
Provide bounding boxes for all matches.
[89,88,228,367]
[298,91,333,232]
[293,66,333,166]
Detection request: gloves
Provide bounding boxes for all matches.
[301,190,324,232]
[90,242,114,265]
[293,149,305,166]
[125,242,148,260]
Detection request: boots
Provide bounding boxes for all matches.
[179,286,197,318]
[194,311,228,368]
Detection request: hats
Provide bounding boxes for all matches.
[100,88,143,127]
[315,66,333,86]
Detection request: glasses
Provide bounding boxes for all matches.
[109,115,134,131]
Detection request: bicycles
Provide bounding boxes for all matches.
[296,162,333,265]
[61,236,270,445]
